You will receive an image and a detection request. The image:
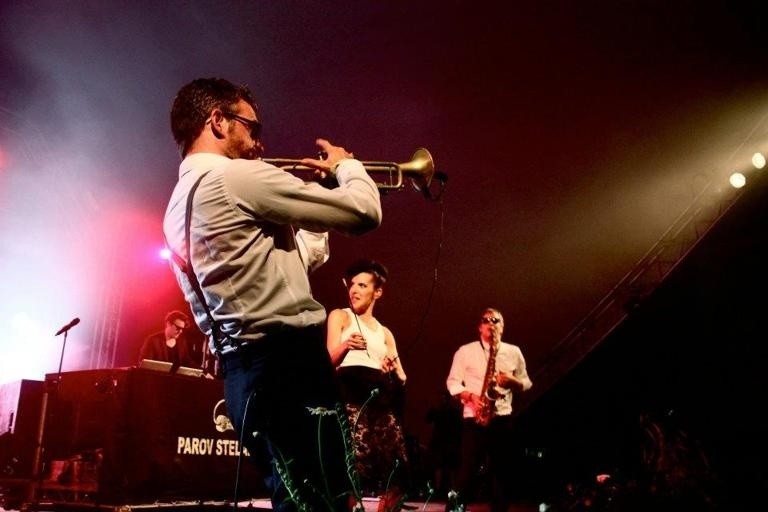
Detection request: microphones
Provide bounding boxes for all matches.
[55,318,81,336]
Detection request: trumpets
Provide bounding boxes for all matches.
[250,147,435,195]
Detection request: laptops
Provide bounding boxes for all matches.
[176,365,203,377]
[140,358,174,372]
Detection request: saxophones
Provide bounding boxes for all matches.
[474,328,503,426]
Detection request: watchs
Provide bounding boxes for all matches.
[330,154,356,177]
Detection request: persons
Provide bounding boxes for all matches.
[137,310,201,368]
[327,255,420,512]
[160,75,386,512]
[446,307,534,512]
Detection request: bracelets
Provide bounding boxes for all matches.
[468,392,474,403]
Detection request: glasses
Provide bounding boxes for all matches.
[206,110,262,137]
[481,317,501,324]
[173,322,184,331]
